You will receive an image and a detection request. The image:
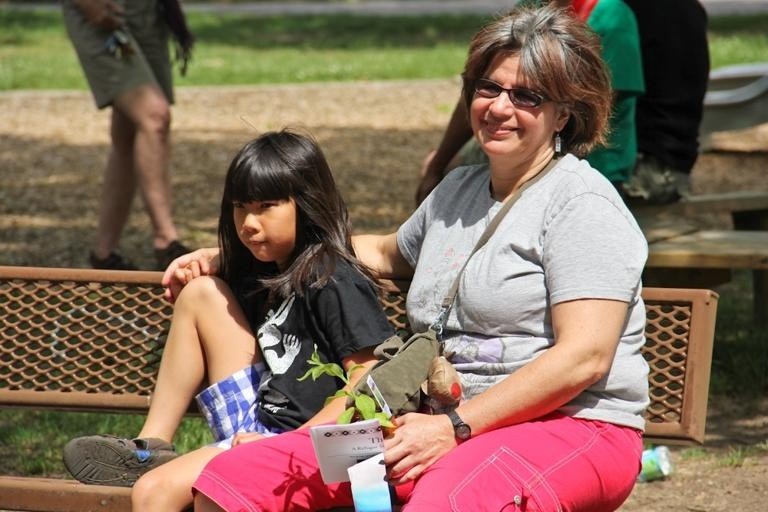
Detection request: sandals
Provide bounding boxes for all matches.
[62,435,176,484]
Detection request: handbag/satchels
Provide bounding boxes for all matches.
[347,332,439,413]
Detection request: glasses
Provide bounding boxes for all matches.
[474,77,562,110]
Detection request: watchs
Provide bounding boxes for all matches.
[444,404,472,443]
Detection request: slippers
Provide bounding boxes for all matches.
[88,253,141,269]
[154,241,192,270]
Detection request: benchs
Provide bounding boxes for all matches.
[626,187,767,269]
[0,266,720,511]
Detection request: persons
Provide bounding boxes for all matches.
[63,128,402,511]
[614,1,712,205]
[414,0,645,213]
[59,0,197,271]
[161,0,649,512]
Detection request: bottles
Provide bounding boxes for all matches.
[636,445,673,482]
